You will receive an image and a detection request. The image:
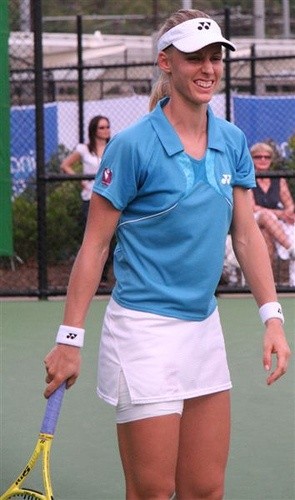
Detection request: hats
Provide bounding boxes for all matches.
[158,17,236,54]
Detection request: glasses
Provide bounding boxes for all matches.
[253,155,271,159]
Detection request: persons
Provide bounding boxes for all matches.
[44,9,291,500]
[60,116,110,286]
[251,143,295,267]
